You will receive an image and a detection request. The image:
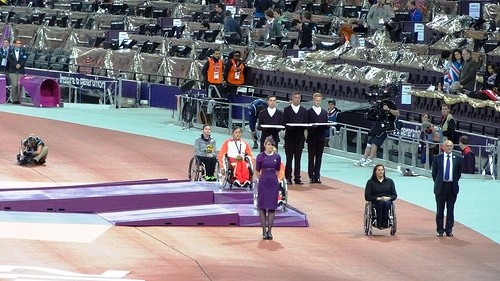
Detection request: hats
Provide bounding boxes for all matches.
[327,99,336,106]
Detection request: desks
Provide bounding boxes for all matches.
[0,0,500,178]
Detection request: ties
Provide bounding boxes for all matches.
[445,154,451,182]
[4,49,7,58]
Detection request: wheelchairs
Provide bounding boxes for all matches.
[254,162,288,212]
[363,200,398,239]
[219,154,255,190]
[188,154,221,183]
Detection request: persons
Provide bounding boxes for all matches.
[306,93,327,183]
[248,97,270,149]
[431,140,462,236]
[195,123,218,181]
[441,48,500,95]
[216,126,253,188]
[212,0,243,44]
[364,164,397,228]
[16,134,48,165]
[201,49,225,98]
[257,96,285,153]
[255,135,286,239]
[0,38,12,102]
[354,97,400,167]
[8,37,27,103]
[283,93,308,184]
[419,104,477,174]
[324,99,342,146]
[253,0,423,48]
[224,49,246,97]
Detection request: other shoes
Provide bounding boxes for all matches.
[242,180,250,187]
[310,177,321,183]
[205,176,217,181]
[294,178,303,184]
[262,231,272,240]
[30,159,37,165]
[286,178,292,185]
[446,232,454,237]
[13,101,20,104]
[436,232,443,237]
[354,158,366,166]
[39,159,46,164]
[360,159,373,167]
[233,180,242,187]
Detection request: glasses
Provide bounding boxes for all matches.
[234,56,240,58]
